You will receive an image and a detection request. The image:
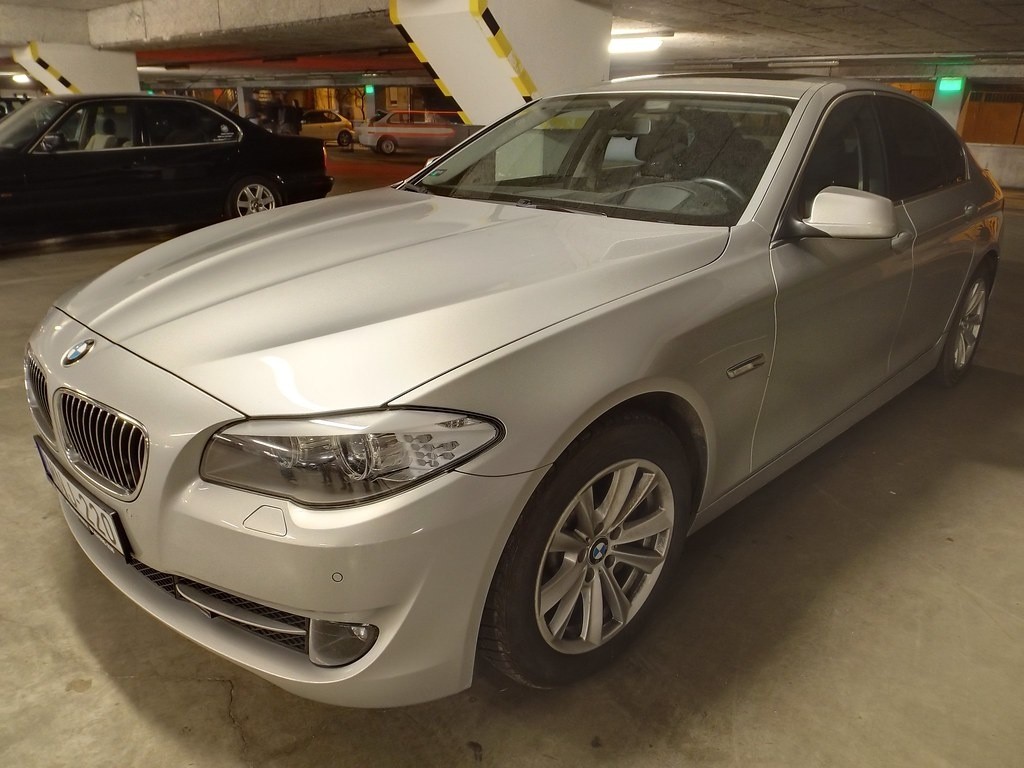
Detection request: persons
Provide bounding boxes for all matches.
[285,98,303,135]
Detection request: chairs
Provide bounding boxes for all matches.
[635,121,688,175]
[84,117,116,149]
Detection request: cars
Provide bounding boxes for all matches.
[358,108,487,156]
[297,108,356,147]
[0,91,337,240]
[20,72,1008,709]
[239,103,303,138]
